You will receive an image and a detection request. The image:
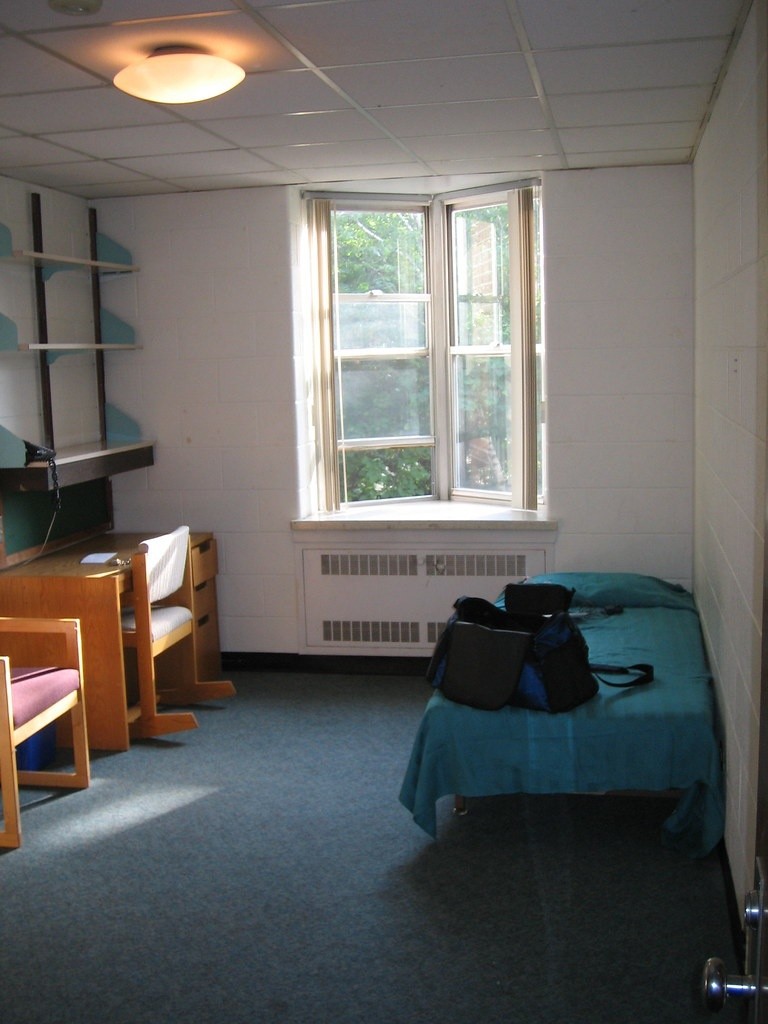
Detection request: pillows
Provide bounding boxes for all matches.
[522,572,680,608]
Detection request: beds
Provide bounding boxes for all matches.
[396,572,723,875]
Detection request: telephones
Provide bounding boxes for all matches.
[23,440,57,462]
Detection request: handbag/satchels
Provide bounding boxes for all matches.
[425,583,654,715]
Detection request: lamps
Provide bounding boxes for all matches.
[113,47,246,104]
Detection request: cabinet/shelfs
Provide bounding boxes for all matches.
[0,193,155,492]
[0,531,219,752]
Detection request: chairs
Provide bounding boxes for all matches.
[0,616,91,847]
[119,525,236,739]
